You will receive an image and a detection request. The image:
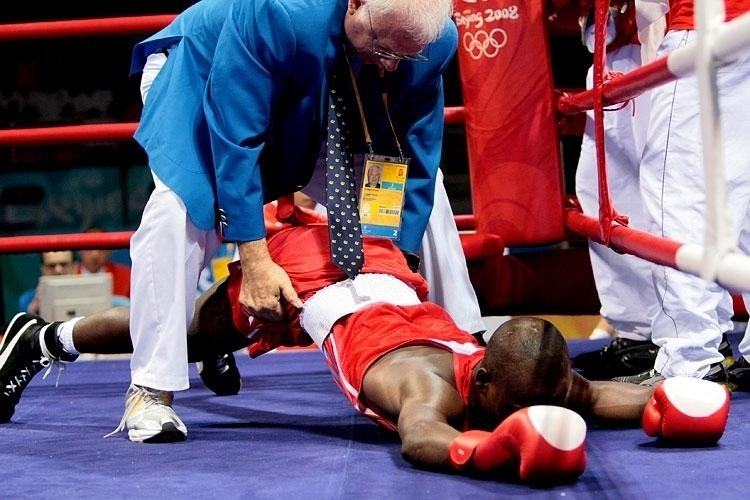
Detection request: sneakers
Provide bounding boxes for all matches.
[0,311,78,424]
[196,353,242,394]
[572,333,750,392]
[125,385,188,442]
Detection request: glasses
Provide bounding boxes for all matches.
[367,6,431,64]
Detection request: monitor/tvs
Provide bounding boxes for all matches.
[38,273,113,323]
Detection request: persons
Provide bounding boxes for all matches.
[0,214,734,493]
[567,0,657,382]
[614,1,748,402]
[122,0,490,443]
[13,250,75,315]
[76,227,131,299]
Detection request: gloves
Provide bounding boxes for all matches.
[642,376,731,443]
[448,406,587,485]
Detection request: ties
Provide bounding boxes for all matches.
[328,68,367,280]
